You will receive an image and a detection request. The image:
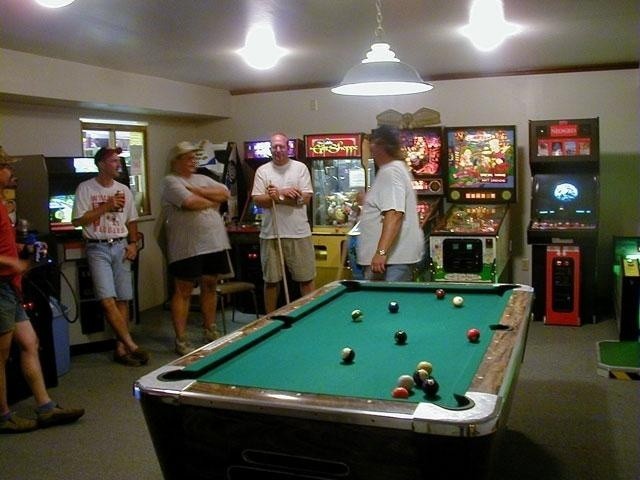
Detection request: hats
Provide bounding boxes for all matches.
[171,142,201,158]
[95,146,122,163]
[0,146,23,165]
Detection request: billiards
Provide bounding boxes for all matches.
[389,301,399,312]
[352,310,364,321]
[467,329,480,341]
[453,296,463,305]
[395,331,407,343]
[341,347,354,362]
[436,288,444,298]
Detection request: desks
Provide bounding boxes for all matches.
[137,280,544,479]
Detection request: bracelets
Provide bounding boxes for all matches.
[131,240,139,246]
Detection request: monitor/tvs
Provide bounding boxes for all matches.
[533,173,597,225]
[444,126,517,202]
[48,194,75,226]
[390,126,443,196]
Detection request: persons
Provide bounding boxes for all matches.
[251,131,313,313]
[160,142,231,355]
[0,145,85,434]
[355,126,425,282]
[72,145,152,368]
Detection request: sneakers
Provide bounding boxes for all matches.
[202,323,222,341]
[36,405,85,427]
[115,347,150,367]
[0,414,40,434]
[174,334,194,355]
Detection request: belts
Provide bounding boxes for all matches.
[89,238,122,243]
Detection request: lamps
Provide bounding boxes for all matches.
[328,2,436,96]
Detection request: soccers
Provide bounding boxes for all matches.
[392,361,439,398]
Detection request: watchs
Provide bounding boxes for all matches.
[279,194,285,203]
[376,249,387,256]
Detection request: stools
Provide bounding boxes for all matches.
[191,254,259,335]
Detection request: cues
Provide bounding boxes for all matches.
[272,198,290,305]
